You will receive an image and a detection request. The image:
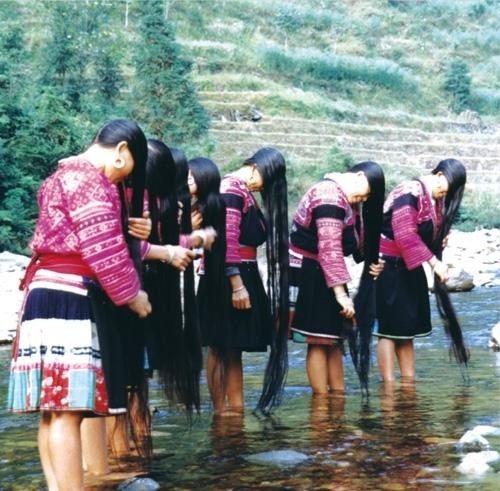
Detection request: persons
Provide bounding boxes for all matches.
[96,139,227,453]
[9,119,151,491]
[197,150,287,414]
[287,162,386,394]
[377,158,467,382]
